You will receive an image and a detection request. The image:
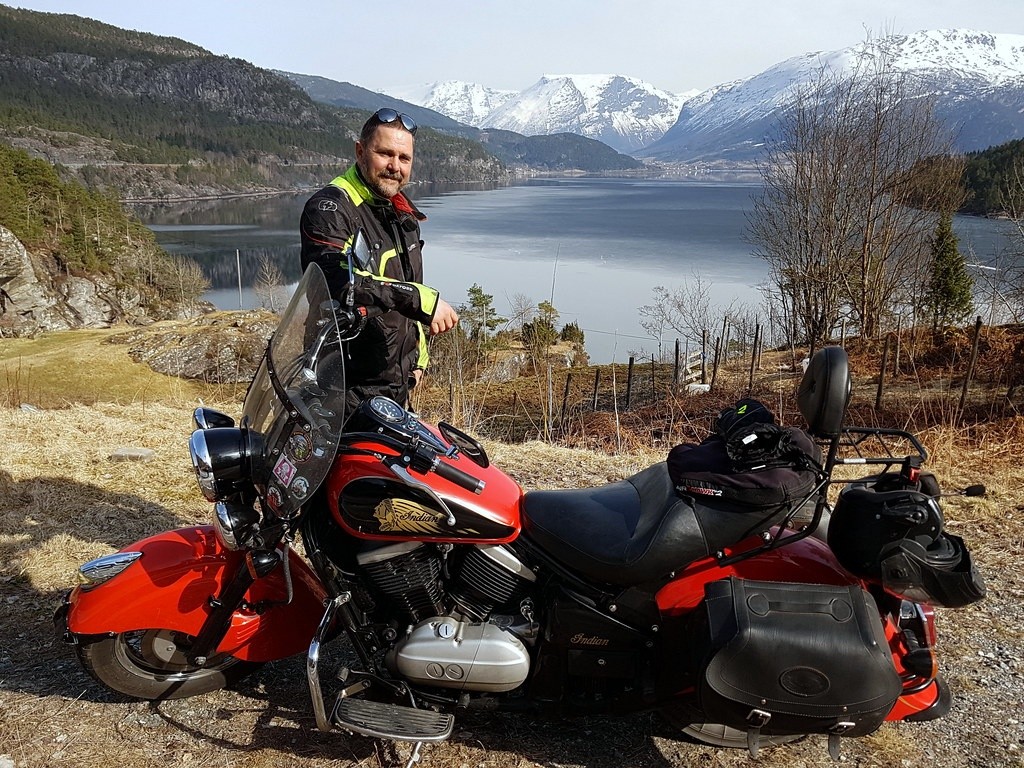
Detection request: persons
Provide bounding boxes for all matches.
[299,105,460,411]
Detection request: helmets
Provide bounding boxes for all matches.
[827,471,966,605]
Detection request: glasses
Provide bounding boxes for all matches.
[376,108,418,135]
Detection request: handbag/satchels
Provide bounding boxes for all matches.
[696,573,903,762]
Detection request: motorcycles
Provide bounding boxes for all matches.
[49,227,988,764]
